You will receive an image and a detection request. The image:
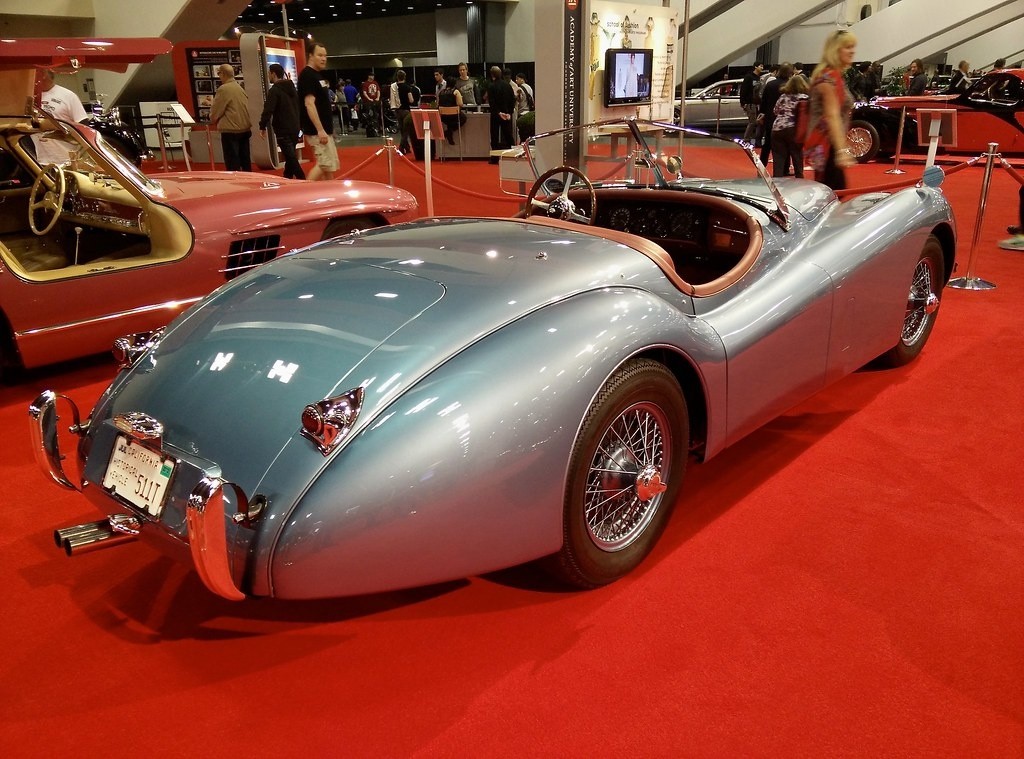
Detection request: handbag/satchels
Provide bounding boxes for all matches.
[350,107,359,120]
[794,98,810,144]
[520,86,534,111]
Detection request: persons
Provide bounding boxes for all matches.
[38,68,91,125]
[209,43,534,183]
[720,27,1023,252]
[625,54,641,98]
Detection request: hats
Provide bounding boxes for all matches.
[516,73,527,82]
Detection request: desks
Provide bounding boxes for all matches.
[436,112,516,161]
[598,120,672,159]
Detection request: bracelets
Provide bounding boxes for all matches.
[833,148,846,154]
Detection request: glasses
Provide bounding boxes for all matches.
[217,71,222,76]
[458,69,467,71]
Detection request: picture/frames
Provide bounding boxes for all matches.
[193,50,244,124]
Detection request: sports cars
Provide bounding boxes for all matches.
[30,116,957,606]
[870,68,1023,156]
[0,115,419,398]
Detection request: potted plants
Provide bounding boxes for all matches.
[517,111,535,145]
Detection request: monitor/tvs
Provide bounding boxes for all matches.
[604,49,653,108]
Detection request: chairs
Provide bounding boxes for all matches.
[1,237,68,273]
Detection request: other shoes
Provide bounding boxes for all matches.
[488,157,499,164]
[999,234,1024,250]
[444,130,455,145]
[399,143,410,155]
[1007,225,1024,235]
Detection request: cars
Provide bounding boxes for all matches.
[842,100,922,165]
[76,116,156,167]
[668,78,810,134]
[924,73,979,95]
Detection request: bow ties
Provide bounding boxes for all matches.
[437,83,443,90]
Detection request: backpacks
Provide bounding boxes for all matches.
[753,75,775,104]
